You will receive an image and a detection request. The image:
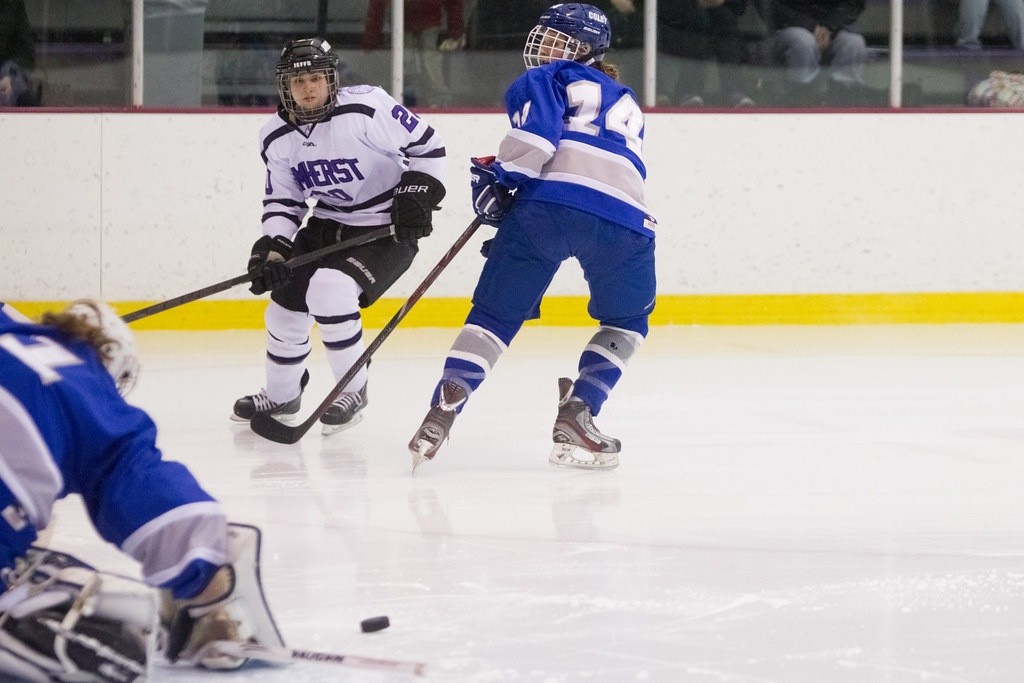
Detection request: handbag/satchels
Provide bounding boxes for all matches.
[966,69,1024,109]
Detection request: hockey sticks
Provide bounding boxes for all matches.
[124,622,424,676]
[249,214,485,445]
[119,222,397,324]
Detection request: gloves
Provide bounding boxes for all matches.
[476,180,510,227]
[249,234,295,295]
[391,170,446,246]
[156,561,247,670]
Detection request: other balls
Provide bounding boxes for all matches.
[361,615,390,633]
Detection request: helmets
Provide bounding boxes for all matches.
[275,39,339,87]
[538,3,611,61]
[65,297,136,394]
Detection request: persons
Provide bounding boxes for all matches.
[362,0,867,109]
[951,0,1024,106]
[409,4,657,475]
[230,38,448,434]
[0,0,43,106]
[0,293,290,683]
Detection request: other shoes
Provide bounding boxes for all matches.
[439,32,467,52]
[674,81,703,108]
[721,85,755,108]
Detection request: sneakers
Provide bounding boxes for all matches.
[320,360,372,436]
[550,376,622,469]
[409,375,470,476]
[230,368,311,423]
